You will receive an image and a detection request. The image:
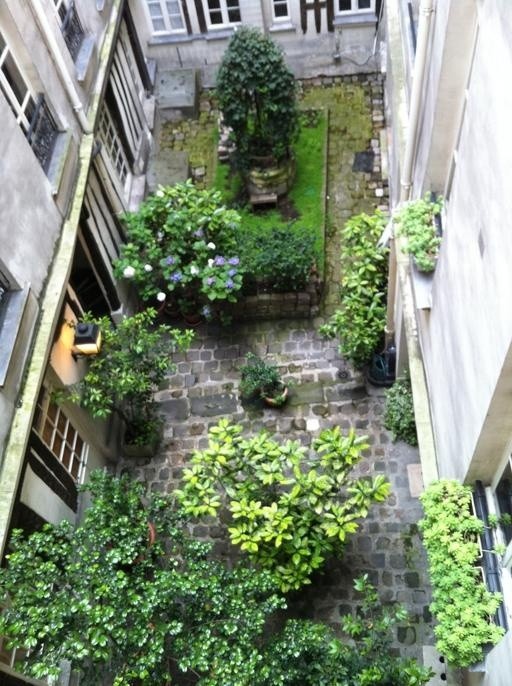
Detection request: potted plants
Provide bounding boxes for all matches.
[215,26,301,197]
[236,352,293,407]
[416,479,506,668]
[50,307,196,457]
[73,467,156,568]
[392,190,444,273]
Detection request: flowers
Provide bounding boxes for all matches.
[112,178,246,328]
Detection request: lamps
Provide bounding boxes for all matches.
[71,323,103,362]
